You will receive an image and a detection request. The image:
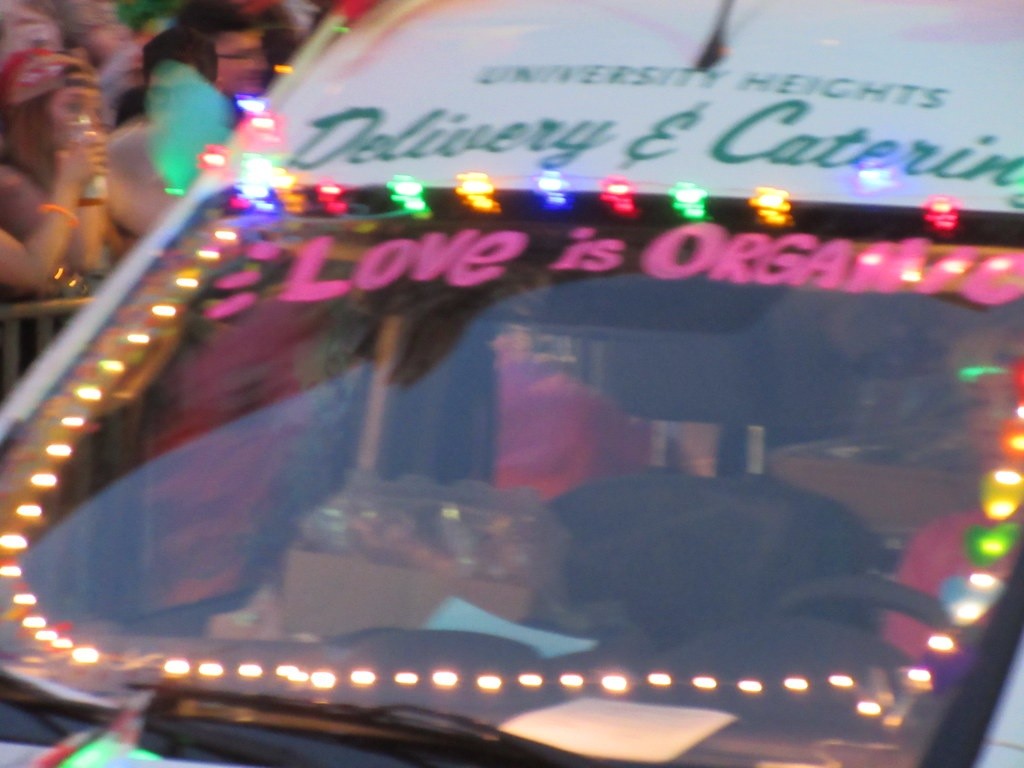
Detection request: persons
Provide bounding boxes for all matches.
[1,0,338,408]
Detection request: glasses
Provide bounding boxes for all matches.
[216,48,273,63]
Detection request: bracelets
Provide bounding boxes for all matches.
[37,204,80,230]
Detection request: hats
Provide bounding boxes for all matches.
[5,51,107,110]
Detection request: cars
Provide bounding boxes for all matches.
[1,2,1023,767]
